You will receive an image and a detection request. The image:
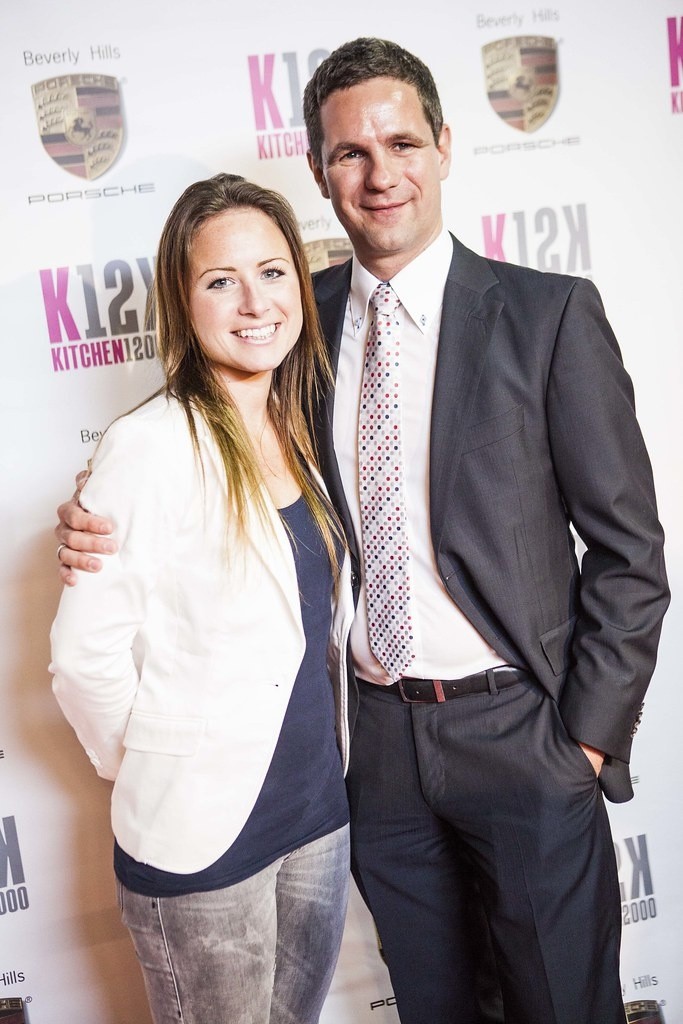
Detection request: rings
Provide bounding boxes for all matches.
[57,544,67,560]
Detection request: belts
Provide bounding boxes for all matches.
[357,666,531,703]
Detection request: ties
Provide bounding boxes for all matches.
[357,283,415,683]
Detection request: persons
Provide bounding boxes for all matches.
[46,172,357,1024]
[55,37,671,1024]
